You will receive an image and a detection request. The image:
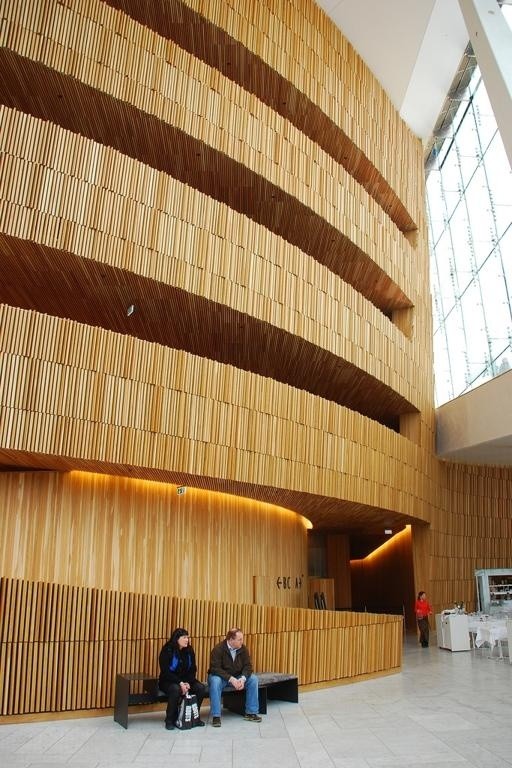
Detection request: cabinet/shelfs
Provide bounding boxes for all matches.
[479,568,512,611]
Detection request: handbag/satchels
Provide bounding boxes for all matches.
[174,691,200,731]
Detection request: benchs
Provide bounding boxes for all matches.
[115,673,298,730]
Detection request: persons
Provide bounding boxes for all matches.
[158,627,207,730]
[414,591,435,649]
[202,627,263,727]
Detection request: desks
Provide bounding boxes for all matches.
[467,614,512,666]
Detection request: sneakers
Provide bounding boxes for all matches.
[198,719,205,726]
[422,642,428,647]
[212,716,222,727]
[166,720,174,731]
[244,714,262,722]
